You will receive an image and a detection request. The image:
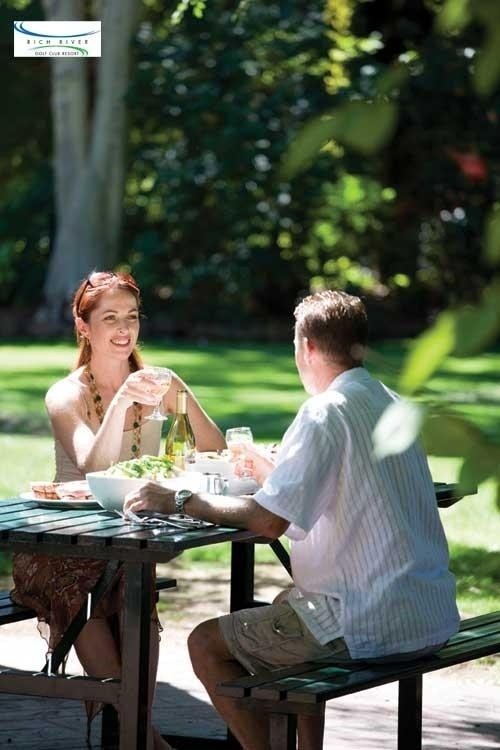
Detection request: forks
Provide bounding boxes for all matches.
[122,509,196,529]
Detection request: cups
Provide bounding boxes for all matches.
[225,426,254,456]
[143,368,172,423]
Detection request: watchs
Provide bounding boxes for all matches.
[174,488,192,515]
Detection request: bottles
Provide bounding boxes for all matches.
[164,389,195,463]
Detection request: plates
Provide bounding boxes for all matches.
[21,491,99,510]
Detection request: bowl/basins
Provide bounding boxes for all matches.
[85,470,188,513]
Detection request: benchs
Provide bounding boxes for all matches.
[214,610,500,750]
[0,571,177,629]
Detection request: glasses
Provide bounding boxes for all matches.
[76,270,137,319]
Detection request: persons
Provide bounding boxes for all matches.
[124,289,460,749]
[14,273,224,749]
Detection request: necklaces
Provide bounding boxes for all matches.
[84,359,143,459]
